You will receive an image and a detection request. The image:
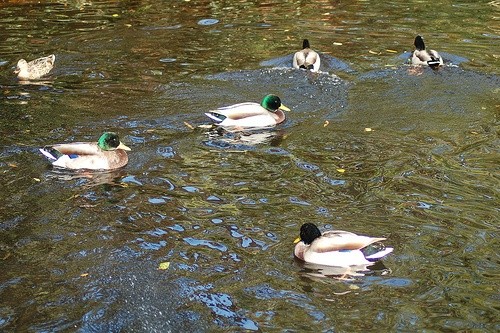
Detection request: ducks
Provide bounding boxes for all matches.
[204,93,292,131]
[293,222,394,265]
[292,39,320,72]
[411,35,444,67]
[13,53,55,80]
[38,132,132,169]
